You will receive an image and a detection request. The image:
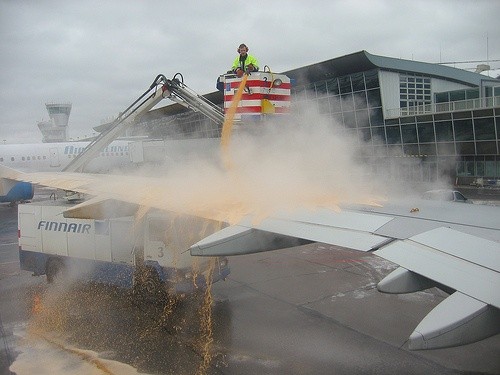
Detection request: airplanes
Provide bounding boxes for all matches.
[0,141,146,199]
[1,165,500,353]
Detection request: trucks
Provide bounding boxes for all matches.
[17,194,233,309]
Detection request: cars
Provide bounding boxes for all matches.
[423,189,473,204]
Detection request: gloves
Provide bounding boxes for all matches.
[237,69,243,78]
[248,64,253,71]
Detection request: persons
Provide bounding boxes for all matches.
[232,44,259,78]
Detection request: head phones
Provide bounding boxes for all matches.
[237,47,249,54]
